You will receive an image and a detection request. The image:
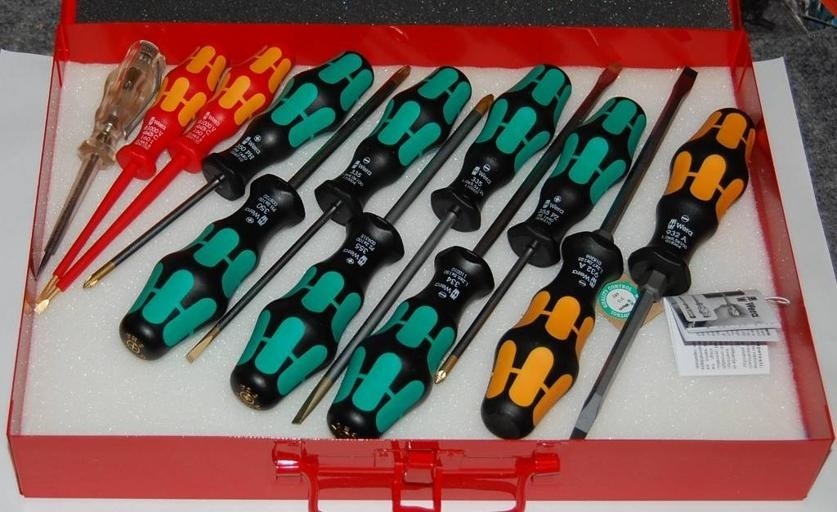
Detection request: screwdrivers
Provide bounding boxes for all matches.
[32,40,756,438]
